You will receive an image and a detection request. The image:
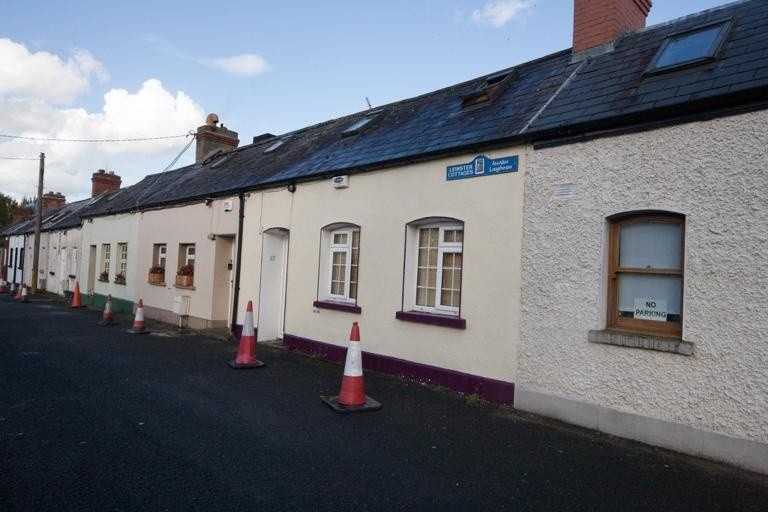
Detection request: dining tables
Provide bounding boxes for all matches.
[100,272,108,281]
[175,263,194,287]
[114,273,123,283]
[148,264,165,283]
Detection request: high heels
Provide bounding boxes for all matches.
[124,299,150,334]
[321,322,382,411]
[0,274,28,302]
[95,293,118,326]
[68,283,85,310]
[227,302,265,368]
